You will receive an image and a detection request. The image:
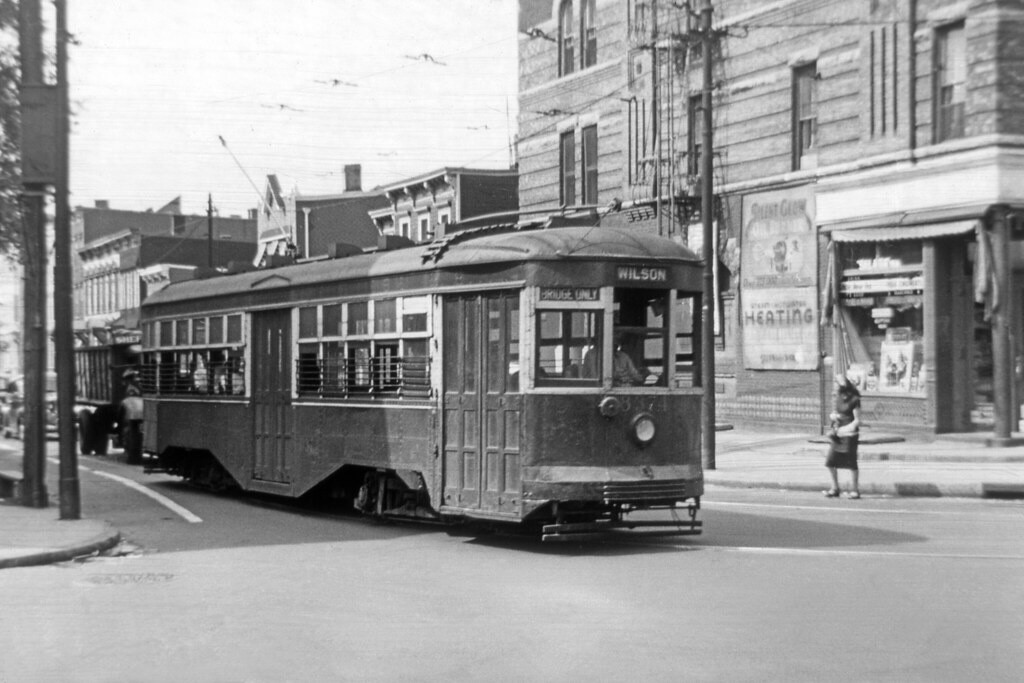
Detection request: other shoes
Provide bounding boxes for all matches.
[822,489,839,497]
[848,490,860,499]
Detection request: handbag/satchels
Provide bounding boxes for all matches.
[830,420,850,453]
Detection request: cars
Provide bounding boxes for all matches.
[6,371,67,443]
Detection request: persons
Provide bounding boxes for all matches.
[581,334,643,387]
[821,373,861,500]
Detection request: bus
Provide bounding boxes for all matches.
[136,223,702,543]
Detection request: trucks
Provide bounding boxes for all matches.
[75,344,145,461]
